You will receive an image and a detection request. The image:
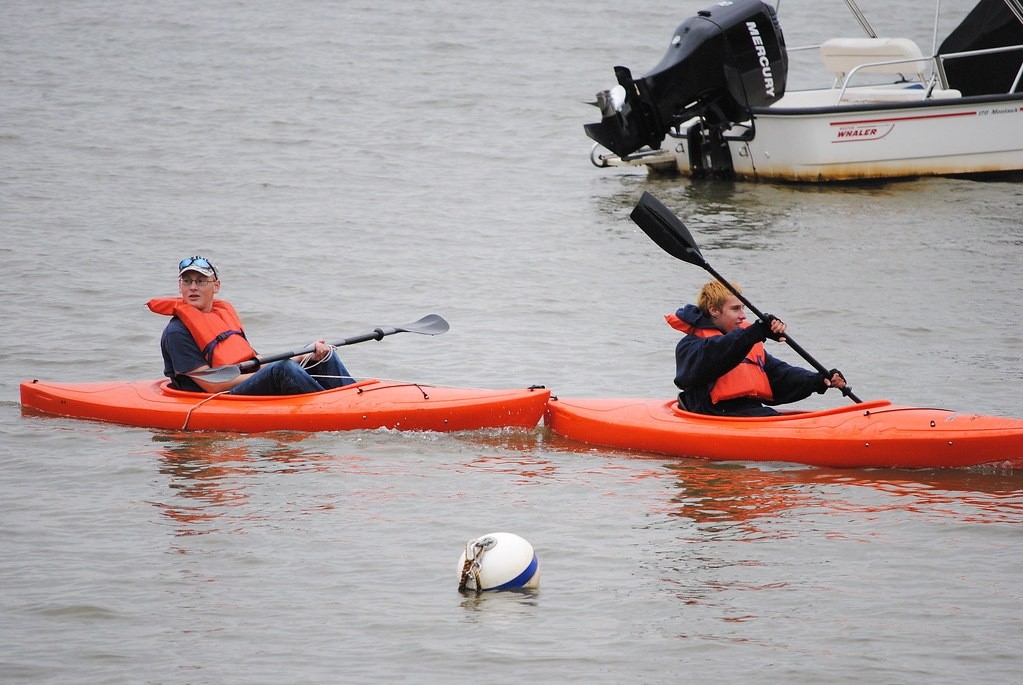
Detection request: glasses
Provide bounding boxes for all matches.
[179,256,217,280]
[179,276,216,286]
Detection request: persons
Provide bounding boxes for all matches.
[664,281,847,416]
[144,259,356,395]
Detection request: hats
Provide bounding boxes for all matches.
[177,256,219,280]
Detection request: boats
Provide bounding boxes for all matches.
[18,373,550,433]
[581,2,1022,185]
[546,387,1023,472]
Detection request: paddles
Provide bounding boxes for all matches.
[627,190,864,405]
[184,313,451,384]
